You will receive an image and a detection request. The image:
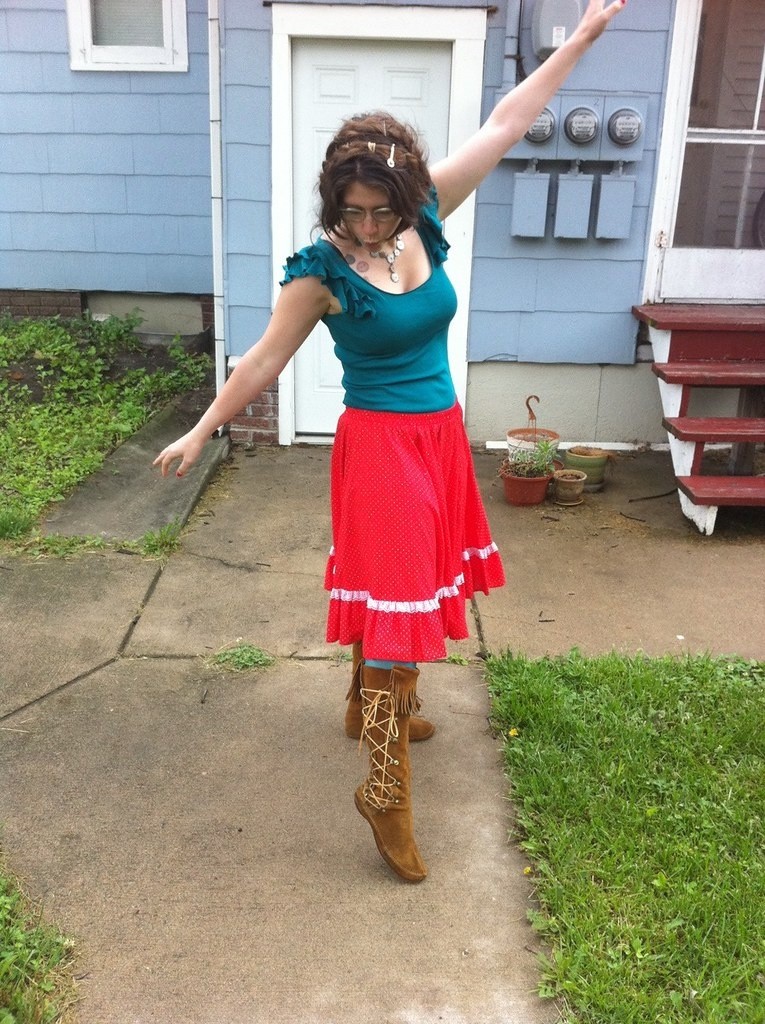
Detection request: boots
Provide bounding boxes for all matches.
[353,664,428,882]
[345,640,436,741]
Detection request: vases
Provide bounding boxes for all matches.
[554,469,587,503]
[507,428,560,461]
[565,446,609,484]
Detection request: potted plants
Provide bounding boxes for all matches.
[495,440,564,507]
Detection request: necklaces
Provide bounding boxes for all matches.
[355,234,405,283]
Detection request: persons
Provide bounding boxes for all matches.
[152,0,628,883]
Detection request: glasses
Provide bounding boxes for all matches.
[335,207,397,223]
